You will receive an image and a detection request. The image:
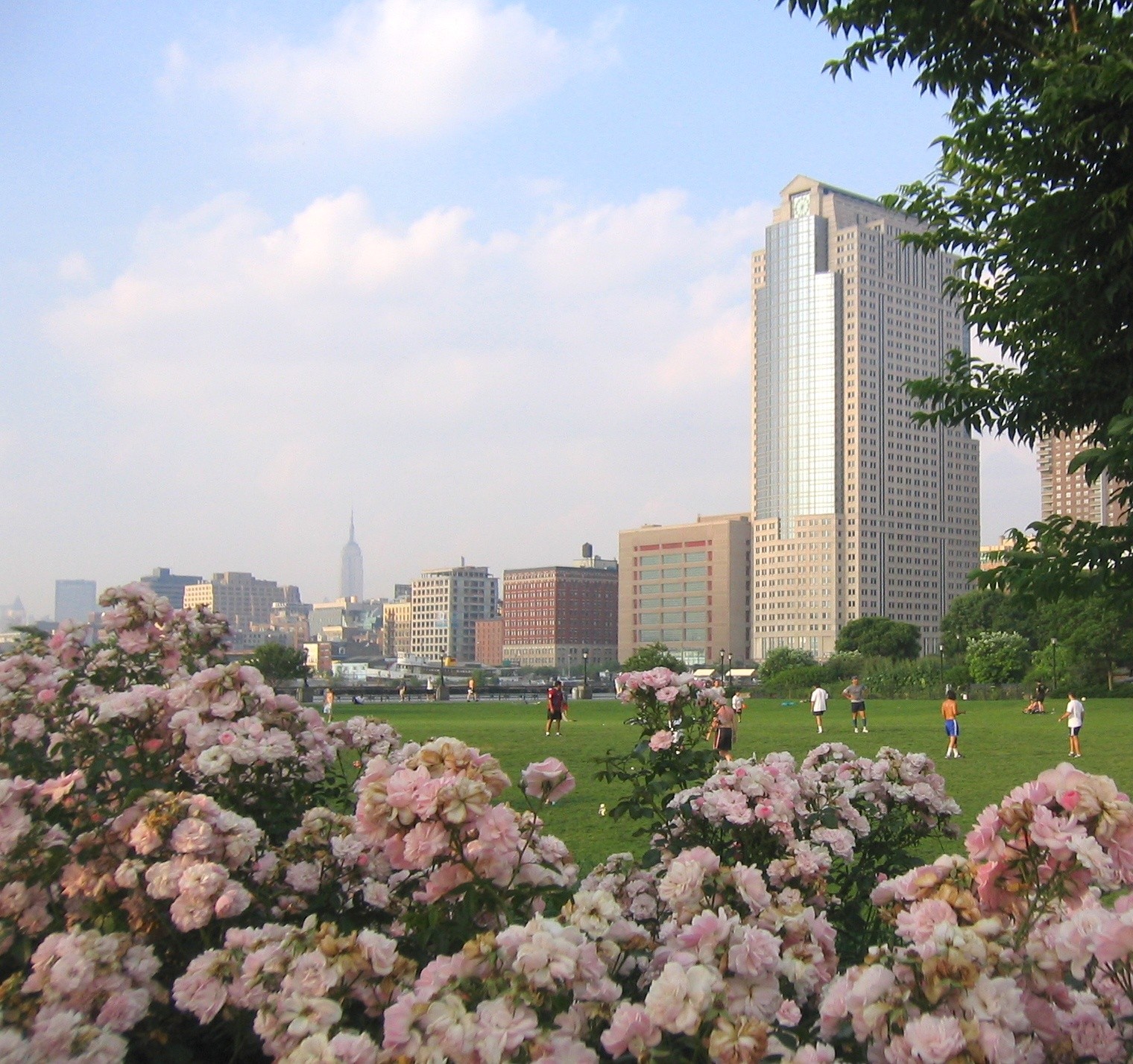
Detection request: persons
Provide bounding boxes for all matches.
[544,682,564,736]
[732,690,743,723]
[841,675,869,734]
[942,690,966,759]
[1058,691,1085,757]
[810,684,828,733]
[396,678,406,702]
[426,676,435,702]
[668,698,686,753]
[352,694,365,704]
[1022,679,1049,715]
[705,696,736,761]
[696,679,726,718]
[323,687,333,724]
[466,677,479,702]
[559,686,567,718]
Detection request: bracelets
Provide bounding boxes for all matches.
[1061,717,1062,720]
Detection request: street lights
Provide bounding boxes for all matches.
[581,652,590,688]
[438,650,448,688]
[1050,635,1060,691]
[727,652,734,687]
[936,644,947,684]
[719,649,728,689]
[301,647,312,684]
